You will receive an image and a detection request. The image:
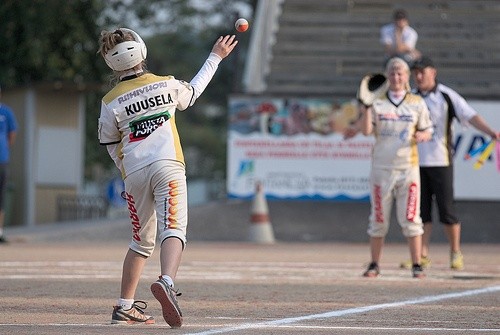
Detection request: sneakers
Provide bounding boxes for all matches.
[151,275,183,327]
[111,304,156,325]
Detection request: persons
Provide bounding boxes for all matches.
[362,57,434,278]
[400,55,500,269]
[98,27,238,328]
[0,104,16,243]
[381,7,422,70]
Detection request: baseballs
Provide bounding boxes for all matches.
[235,19,248,32]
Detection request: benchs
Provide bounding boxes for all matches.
[266,0,500,99]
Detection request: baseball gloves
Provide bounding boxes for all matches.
[357,73,390,108]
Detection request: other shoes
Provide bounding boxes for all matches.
[450,249,463,270]
[0,235,8,243]
[401,255,432,269]
[412,265,426,278]
[363,261,384,277]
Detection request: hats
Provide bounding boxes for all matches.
[410,56,436,70]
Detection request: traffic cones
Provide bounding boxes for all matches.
[245,180,277,245]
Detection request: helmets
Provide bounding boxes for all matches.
[102,28,147,71]
